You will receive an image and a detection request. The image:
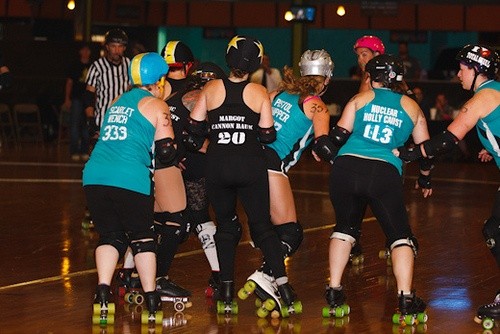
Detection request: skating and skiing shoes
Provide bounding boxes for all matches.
[393,289,428,325]
[157,276,193,311]
[117,266,135,297]
[349,242,364,265]
[379,248,391,266]
[393,324,427,334]
[215,279,238,314]
[216,314,238,334]
[278,282,303,317]
[205,271,221,297]
[323,286,350,317]
[322,316,349,334]
[473,301,500,330]
[239,269,281,319]
[92,283,115,324]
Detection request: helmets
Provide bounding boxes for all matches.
[299,48,335,76]
[128,53,169,85]
[226,35,263,72]
[457,44,500,77]
[354,36,384,53]
[105,28,129,45]
[161,41,195,67]
[364,54,404,83]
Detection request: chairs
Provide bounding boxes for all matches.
[0,103,71,153]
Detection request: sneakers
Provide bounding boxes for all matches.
[141,290,163,324]
[124,266,144,305]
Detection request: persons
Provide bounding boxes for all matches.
[246,48,339,311]
[312,54,434,314]
[60,42,97,160]
[84,28,131,139]
[182,34,298,306]
[392,43,500,320]
[350,35,415,256]
[413,88,455,120]
[250,55,283,94]
[83,52,177,311]
[116,41,242,300]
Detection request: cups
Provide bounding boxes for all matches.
[430,109,437,120]
